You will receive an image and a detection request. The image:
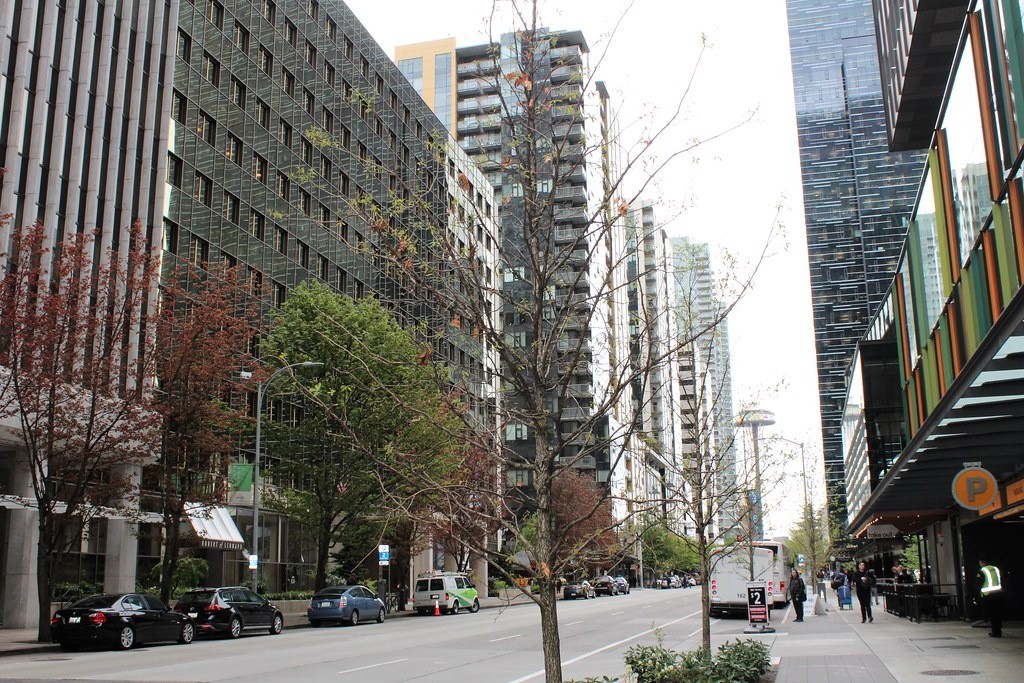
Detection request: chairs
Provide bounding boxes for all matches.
[233,595,241,602]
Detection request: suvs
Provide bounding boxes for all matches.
[173,585,285,639]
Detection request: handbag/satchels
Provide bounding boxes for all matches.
[866,571,877,587]
[801,592,807,601]
[831,573,844,589]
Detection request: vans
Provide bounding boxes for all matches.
[411,570,480,615]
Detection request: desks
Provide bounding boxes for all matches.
[886,592,954,620]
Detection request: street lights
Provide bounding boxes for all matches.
[250,361,323,593]
[755,437,817,593]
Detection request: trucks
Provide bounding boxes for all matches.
[704,546,774,621]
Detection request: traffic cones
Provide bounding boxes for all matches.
[433,600,442,616]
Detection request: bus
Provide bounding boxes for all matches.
[734,541,800,607]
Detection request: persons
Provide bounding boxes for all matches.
[891,565,915,583]
[869,569,880,605]
[975,558,1005,637]
[789,569,805,622]
[683,575,686,589]
[665,576,671,587]
[851,562,876,623]
[628,576,637,588]
[557,580,562,594]
[835,568,852,607]
[688,575,691,588]
[817,570,824,582]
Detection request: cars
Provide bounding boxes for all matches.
[51,593,197,649]
[563,575,630,600]
[307,585,387,625]
[656,572,701,589]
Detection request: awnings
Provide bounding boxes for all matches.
[182,503,245,551]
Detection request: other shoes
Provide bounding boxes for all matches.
[869,617,874,622]
[988,632,1003,638]
[793,618,803,623]
[861,618,866,624]
